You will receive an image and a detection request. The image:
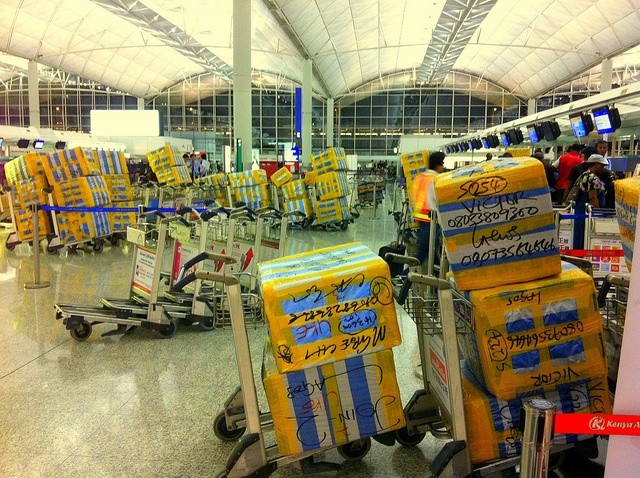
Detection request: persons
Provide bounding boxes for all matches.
[487,153,492,159]
[188,154,203,179]
[216,161,220,171]
[562,146,614,210]
[596,141,612,171]
[183,154,189,163]
[200,154,210,177]
[555,144,584,200]
[130,168,142,183]
[562,154,609,208]
[371,159,396,175]
[410,152,445,276]
[552,145,571,172]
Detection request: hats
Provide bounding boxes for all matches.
[587,154,609,165]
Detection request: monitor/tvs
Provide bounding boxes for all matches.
[55,142,65,149]
[468,140,475,150]
[507,128,523,145]
[33,141,44,149]
[17,140,29,148]
[569,111,594,138]
[458,144,463,152]
[449,146,454,153]
[487,135,499,148]
[461,142,469,151]
[526,123,544,144]
[500,132,511,146]
[0,138,3,148]
[541,121,561,141]
[472,139,482,150]
[452,145,459,153]
[591,105,621,135]
[481,137,490,149]
[446,147,450,154]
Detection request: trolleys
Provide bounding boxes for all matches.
[177,252,406,478]
[53,204,289,340]
